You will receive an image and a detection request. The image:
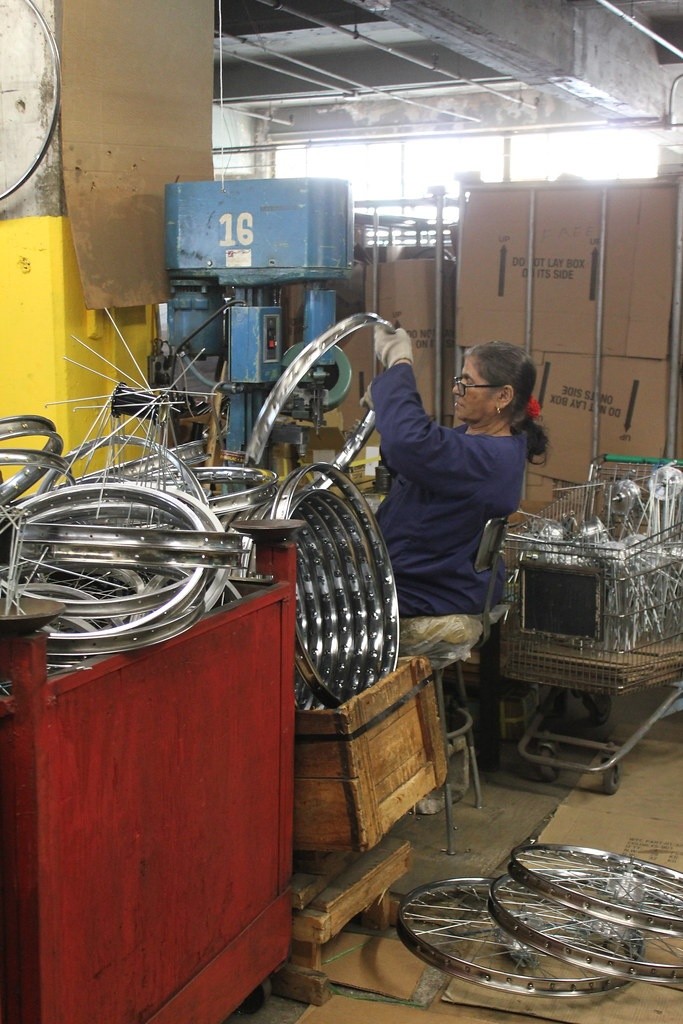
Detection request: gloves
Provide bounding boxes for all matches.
[359,381,375,411]
[373,326,413,368]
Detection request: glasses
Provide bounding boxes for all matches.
[454,376,505,397]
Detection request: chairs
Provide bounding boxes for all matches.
[397,516,510,811]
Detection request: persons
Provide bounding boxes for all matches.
[358,321,549,620]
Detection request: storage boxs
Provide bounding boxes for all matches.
[455,173,678,360]
[0,518,311,1024]
[294,655,447,854]
[366,258,455,416]
[465,349,683,500]
[295,426,382,484]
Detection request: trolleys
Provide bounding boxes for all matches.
[501,453,683,795]
